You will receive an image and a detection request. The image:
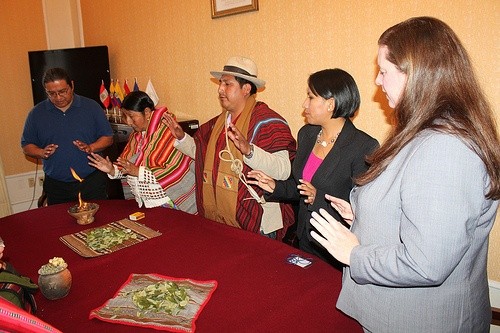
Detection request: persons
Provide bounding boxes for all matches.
[162,57,297,243]
[87,91,199,216]
[246,69,380,269]
[20,67,114,206]
[310,16,500,333]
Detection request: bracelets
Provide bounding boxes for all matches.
[245,144,254,159]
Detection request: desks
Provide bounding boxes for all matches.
[0,199,364,333]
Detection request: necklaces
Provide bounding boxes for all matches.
[317,129,340,148]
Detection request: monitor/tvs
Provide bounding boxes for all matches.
[28,45,112,110]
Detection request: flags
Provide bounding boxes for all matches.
[100,81,159,111]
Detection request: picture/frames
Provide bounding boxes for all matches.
[210,0,259,20]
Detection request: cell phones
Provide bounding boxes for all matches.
[284,253,313,269]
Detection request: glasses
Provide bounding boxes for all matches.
[46,85,70,98]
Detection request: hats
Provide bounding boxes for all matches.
[209,57,265,88]
